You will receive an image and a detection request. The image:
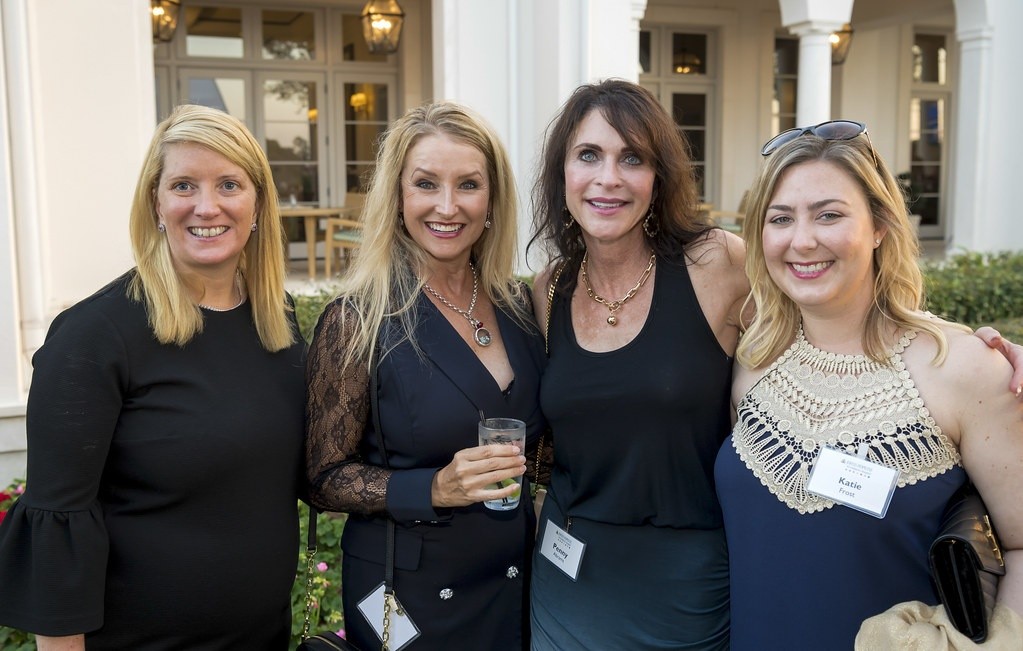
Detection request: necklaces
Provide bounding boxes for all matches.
[582,248,657,325]
[416,262,493,348]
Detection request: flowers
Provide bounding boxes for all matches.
[0,482,23,523]
[297,552,347,641]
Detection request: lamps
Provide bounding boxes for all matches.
[831,23,856,65]
[152,0,183,43]
[348,93,369,112]
[358,0,405,55]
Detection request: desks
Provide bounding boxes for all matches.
[276,200,362,281]
[690,203,714,215]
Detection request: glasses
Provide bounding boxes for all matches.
[760,120,879,170]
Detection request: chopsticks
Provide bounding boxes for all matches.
[477,407,509,504]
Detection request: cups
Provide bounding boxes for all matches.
[475,415,528,511]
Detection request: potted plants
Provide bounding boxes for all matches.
[895,172,923,239]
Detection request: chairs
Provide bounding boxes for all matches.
[708,189,751,236]
[325,213,364,279]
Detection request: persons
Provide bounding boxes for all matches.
[1,103,325,651]
[303,101,545,650]
[522,78,1023,650]
[715,121,1022,650]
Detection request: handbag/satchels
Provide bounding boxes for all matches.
[293,461,396,651]
[928,479,1005,644]
